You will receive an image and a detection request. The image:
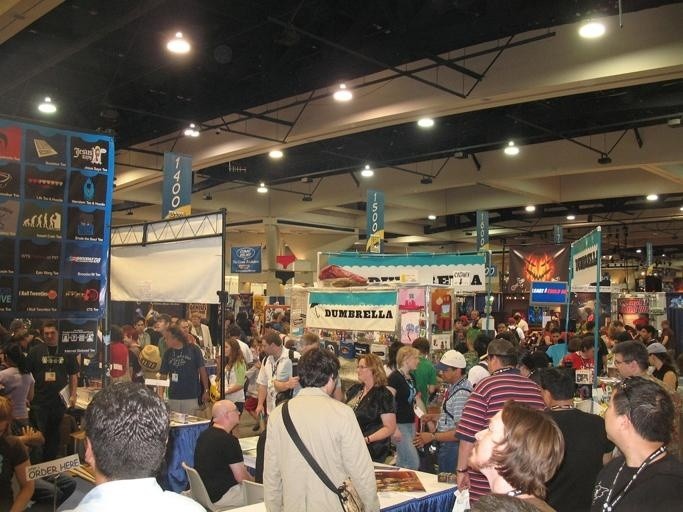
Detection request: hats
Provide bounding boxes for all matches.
[646,342,667,353]
[433,350,467,371]
[339,481,363,511]
[479,338,516,360]
[508,318,516,324]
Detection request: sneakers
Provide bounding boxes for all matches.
[254,420,259,431]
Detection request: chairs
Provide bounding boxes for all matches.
[181,462,238,511]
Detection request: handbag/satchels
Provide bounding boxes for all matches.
[365,426,391,462]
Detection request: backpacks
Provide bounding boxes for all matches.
[508,326,520,342]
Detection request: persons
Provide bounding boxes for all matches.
[0,303,683,511]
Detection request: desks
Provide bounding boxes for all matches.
[76,398,211,494]
[204,362,217,375]
[339,363,393,384]
[225,436,457,512]
